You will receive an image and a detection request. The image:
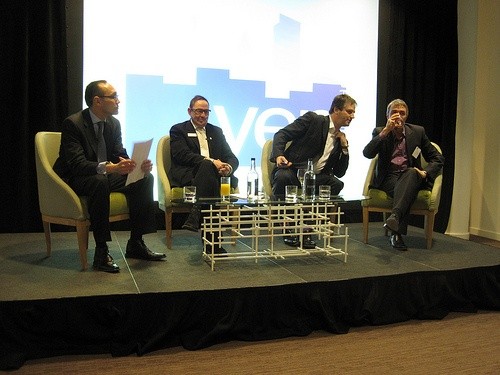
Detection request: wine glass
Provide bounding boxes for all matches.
[297,168,308,189]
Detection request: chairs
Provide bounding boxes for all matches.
[262,141,329,244]
[35,131,130,271]
[158,136,240,249]
[361,143,444,249]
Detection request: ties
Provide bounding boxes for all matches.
[197,128,207,158]
[97,122,107,162]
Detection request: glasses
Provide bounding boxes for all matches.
[99,95,119,99]
[190,109,212,114]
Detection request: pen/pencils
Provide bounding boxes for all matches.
[118,156,137,168]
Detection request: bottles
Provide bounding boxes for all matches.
[302,158,316,200]
[246,158,259,198]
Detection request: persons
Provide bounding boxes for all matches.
[270,94,357,248]
[363,99,444,250]
[52,80,167,273]
[170,95,239,258]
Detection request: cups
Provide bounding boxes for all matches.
[318,185,331,201]
[182,186,196,199]
[395,117,405,128]
[220,177,231,200]
[285,185,298,201]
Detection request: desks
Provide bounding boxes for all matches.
[169,196,369,247]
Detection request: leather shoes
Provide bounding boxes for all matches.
[282,228,297,245]
[182,208,200,232]
[299,230,316,248]
[201,232,227,256]
[93,249,120,271]
[389,234,407,249]
[384,212,399,231]
[126,239,166,260]
